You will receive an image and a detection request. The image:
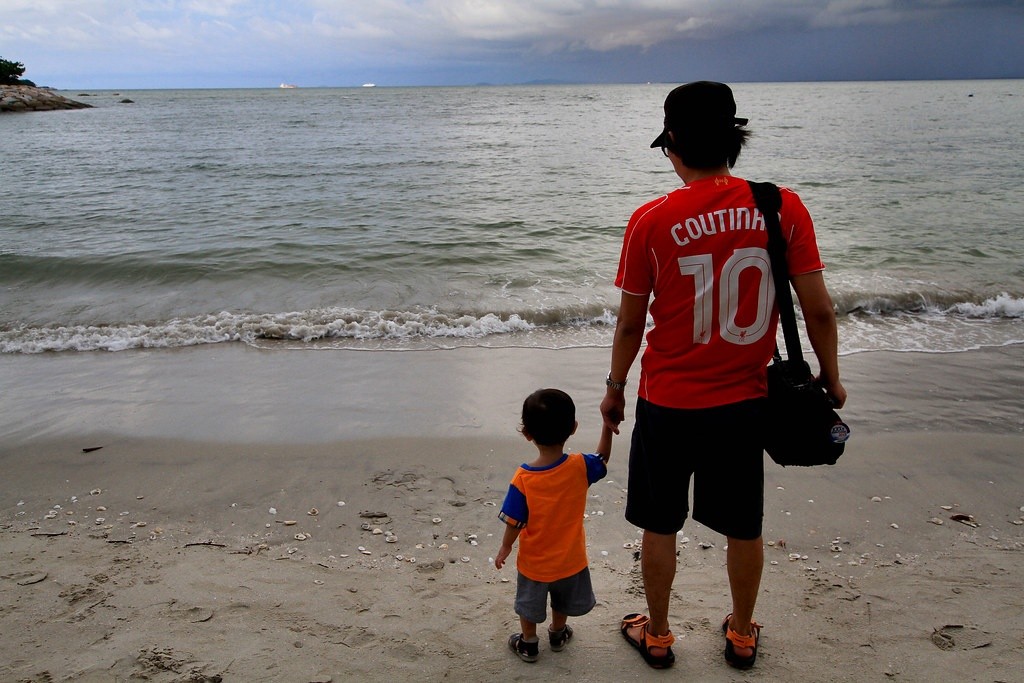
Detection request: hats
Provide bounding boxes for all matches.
[650,81,749,149]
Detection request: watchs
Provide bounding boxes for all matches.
[606,370,628,389]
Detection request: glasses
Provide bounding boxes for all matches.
[662,146,669,157]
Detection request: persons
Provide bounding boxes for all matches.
[494,386,614,662]
[599,78,847,672]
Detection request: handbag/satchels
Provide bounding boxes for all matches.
[763,359,850,468]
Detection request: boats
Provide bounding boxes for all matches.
[280,83,296,89]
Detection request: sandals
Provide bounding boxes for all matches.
[548,622,573,652]
[621,613,676,670]
[723,612,764,671]
[508,632,540,663]
